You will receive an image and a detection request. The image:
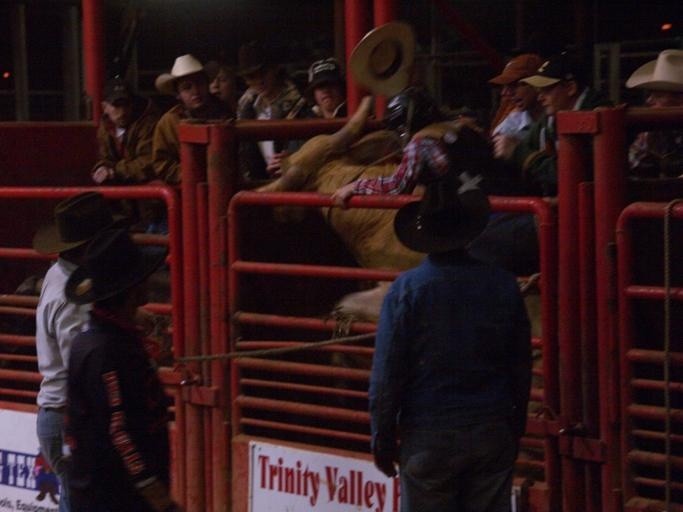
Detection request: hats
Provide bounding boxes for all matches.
[233,45,266,76]
[98,80,127,105]
[65,229,169,303]
[521,60,573,88]
[156,55,210,93]
[626,50,683,90]
[394,180,489,254]
[33,191,128,255]
[348,21,421,96]
[308,58,337,89]
[487,52,543,84]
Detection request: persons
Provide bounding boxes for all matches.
[60,223,180,512]
[92,53,682,212]
[368,180,532,512]
[34,192,127,511]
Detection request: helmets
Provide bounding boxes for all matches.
[384,86,437,131]
